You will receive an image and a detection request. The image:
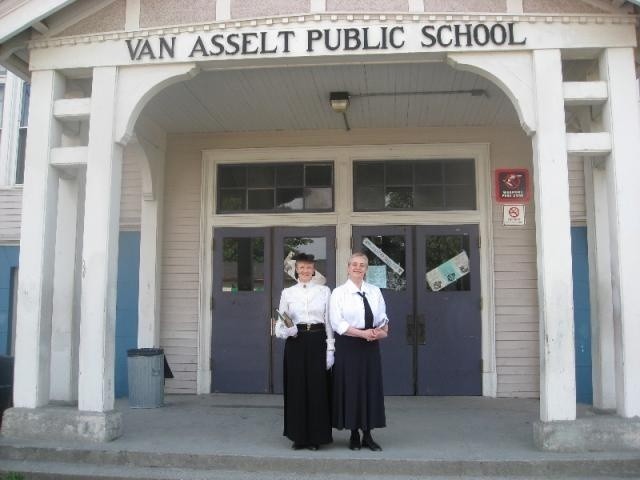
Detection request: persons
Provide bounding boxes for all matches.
[274,253,336,452]
[329,252,389,452]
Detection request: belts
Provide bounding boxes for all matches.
[296,323,323,332]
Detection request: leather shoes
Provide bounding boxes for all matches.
[349,433,384,453]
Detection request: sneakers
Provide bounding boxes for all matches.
[290,440,320,452]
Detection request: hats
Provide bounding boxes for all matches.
[289,252,317,264]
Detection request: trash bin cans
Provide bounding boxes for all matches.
[126,348,165,409]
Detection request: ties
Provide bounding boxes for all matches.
[356,291,375,330]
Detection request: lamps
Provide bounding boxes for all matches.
[330,92,349,112]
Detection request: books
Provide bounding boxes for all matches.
[275,309,299,339]
[374,317,389,329]
